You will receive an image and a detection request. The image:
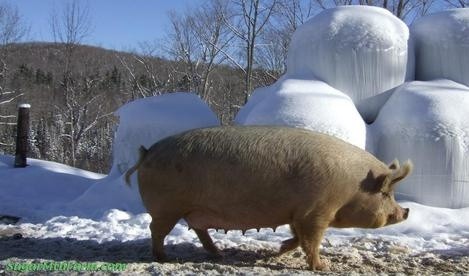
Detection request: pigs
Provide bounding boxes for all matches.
[125,125,413,270]
